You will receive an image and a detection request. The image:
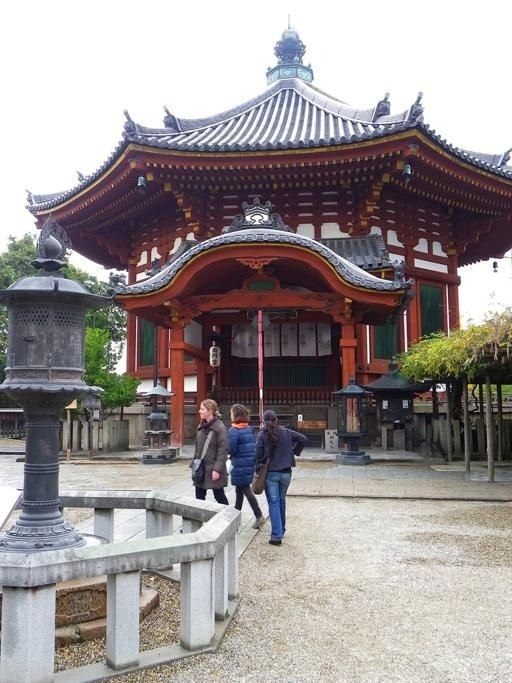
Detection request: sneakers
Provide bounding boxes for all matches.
[269,539,281,544]
[252,516,266,529]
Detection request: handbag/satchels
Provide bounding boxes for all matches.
[190,458,205,486]
[251,462,269,494]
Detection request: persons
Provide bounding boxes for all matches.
[253,410,309,546]
[225,404,267,528]
[188,397,230,534]
